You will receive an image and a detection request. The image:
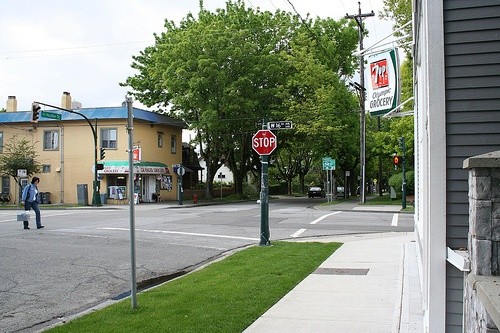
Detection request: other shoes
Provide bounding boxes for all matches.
[24,227,30,229]
[37,225,44,229]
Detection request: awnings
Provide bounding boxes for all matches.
[92,160,167,175]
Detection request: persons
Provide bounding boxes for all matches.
[21,177,45,229]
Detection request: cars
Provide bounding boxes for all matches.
[308,187,327,198]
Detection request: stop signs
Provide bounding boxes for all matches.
[251,130,277,156]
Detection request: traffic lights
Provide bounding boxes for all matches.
[97,164,103,170]
[32,102,41,124]
[399,138,403,150]
[394,156,403,165]
[100,147,106,160]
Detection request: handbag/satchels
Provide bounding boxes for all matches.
[26,185,31,200]
[17,205,31,221]
